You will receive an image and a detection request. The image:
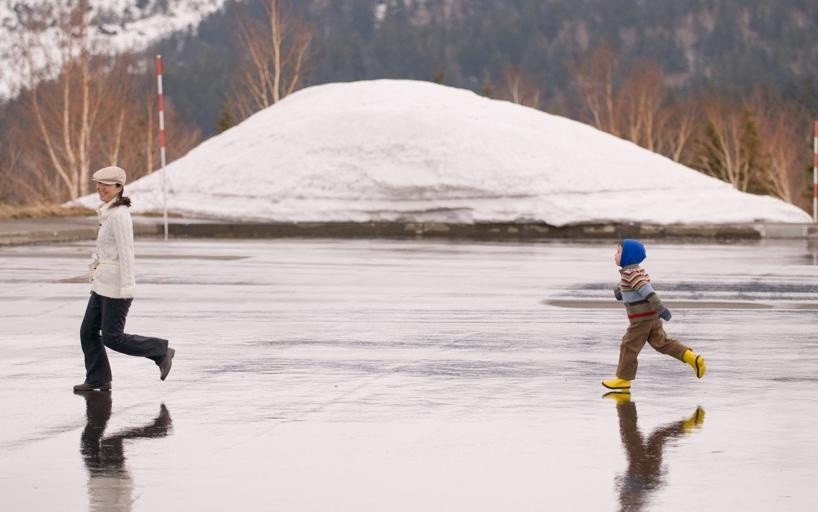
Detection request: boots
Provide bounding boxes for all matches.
[683,350,705,378]
[683,407,705,429]
[603,392,632,402]
[602,377,631,389]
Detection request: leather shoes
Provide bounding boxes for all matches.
[155,404,173,432]
[158,348,174,380]
[74,379,111,391]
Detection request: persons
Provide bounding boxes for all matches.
[72,163,177,391]
[71,392,177,512]
[600,237,707,390]
[600,390,706,512]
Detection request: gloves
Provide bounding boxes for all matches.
[657,307,672,321]
[614,288,622,299]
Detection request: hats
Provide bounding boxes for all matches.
[91,166,126,186]
